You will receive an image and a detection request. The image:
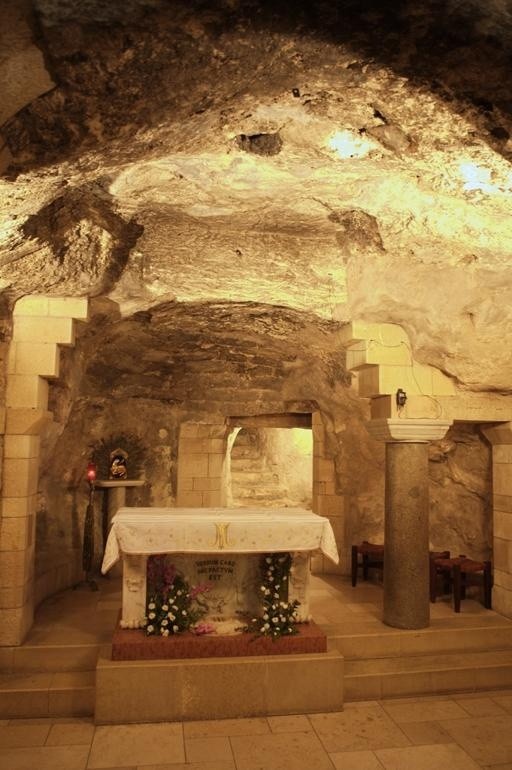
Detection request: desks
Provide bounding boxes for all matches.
[101,506,339,630]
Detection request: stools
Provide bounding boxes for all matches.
[352,540,491,614]
